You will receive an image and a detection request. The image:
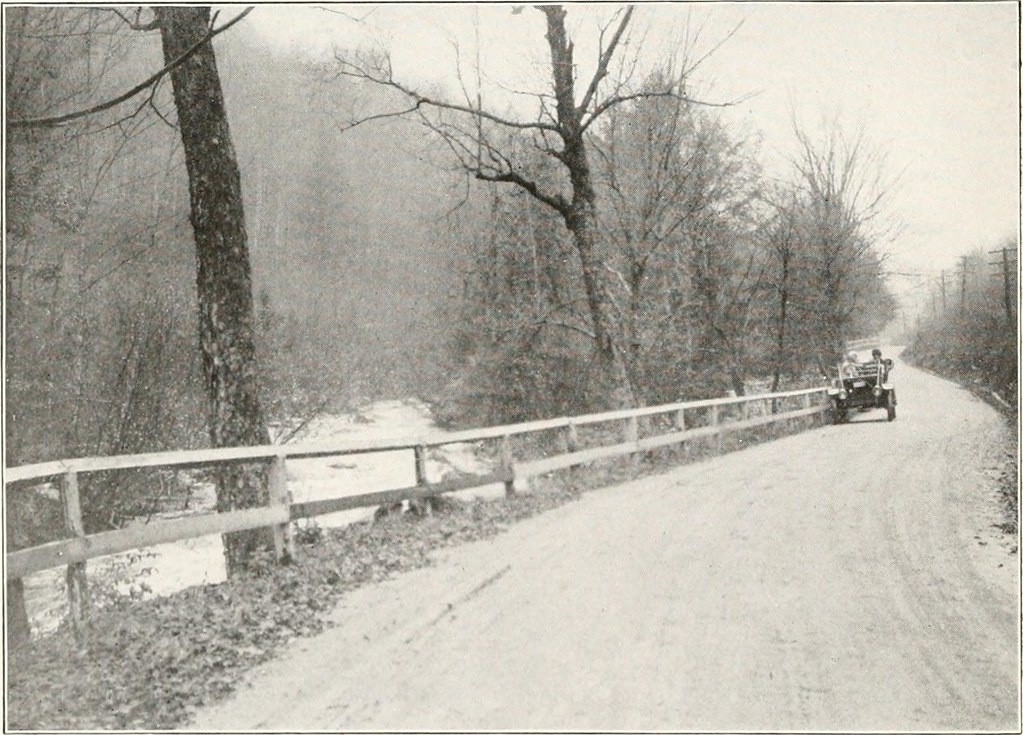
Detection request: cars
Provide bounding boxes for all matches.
[827,362,897,423]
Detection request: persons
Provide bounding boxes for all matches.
[841,349,894,391]
